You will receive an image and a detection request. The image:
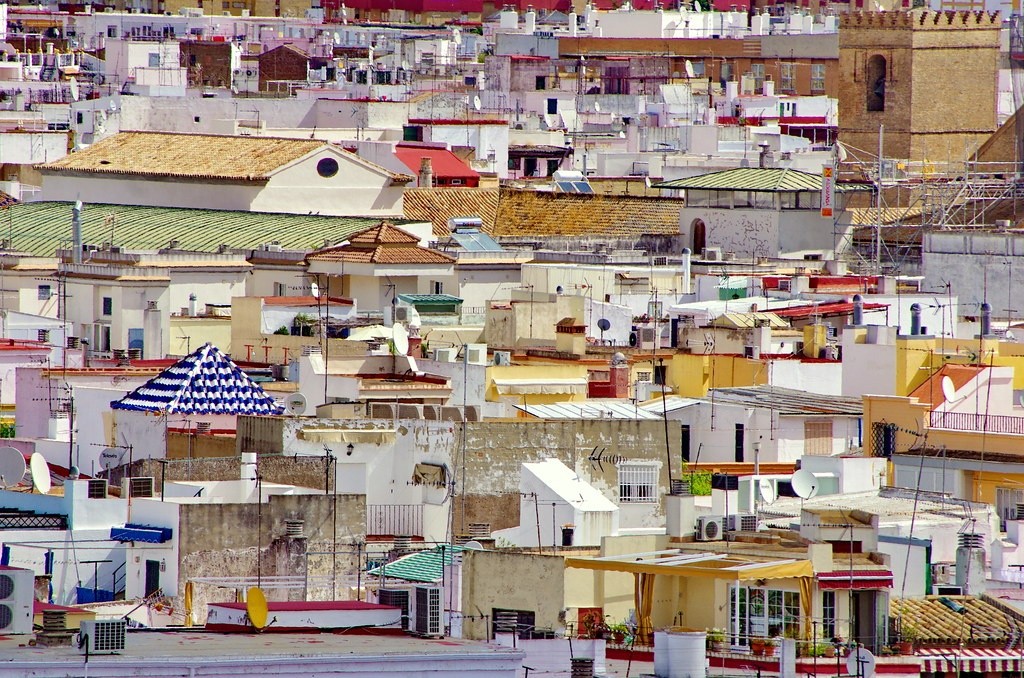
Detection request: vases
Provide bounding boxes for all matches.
[712,641,724,651]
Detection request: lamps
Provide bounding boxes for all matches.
[160,561,166,573]
[347,443,354,456]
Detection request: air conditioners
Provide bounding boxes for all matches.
[79,478,108,499]
[778,280,792,292]
[120,477,155,499]
[378,588,412,632]
[628,331,637,348]
[694,514,757,541]
[0,569,33,636]
[826,347,839,361]
[411,584,445,639]
[743,345,760,360]
[701,247,720,261]
[234,68,257,78]
[513,120,526,130]
[79,620,127,655]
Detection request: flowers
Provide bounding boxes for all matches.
[706,627,727,640]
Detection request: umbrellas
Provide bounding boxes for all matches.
[109,342,284,480]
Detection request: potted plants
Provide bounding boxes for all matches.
[610,626,629,644]
[750,627,915,659]
